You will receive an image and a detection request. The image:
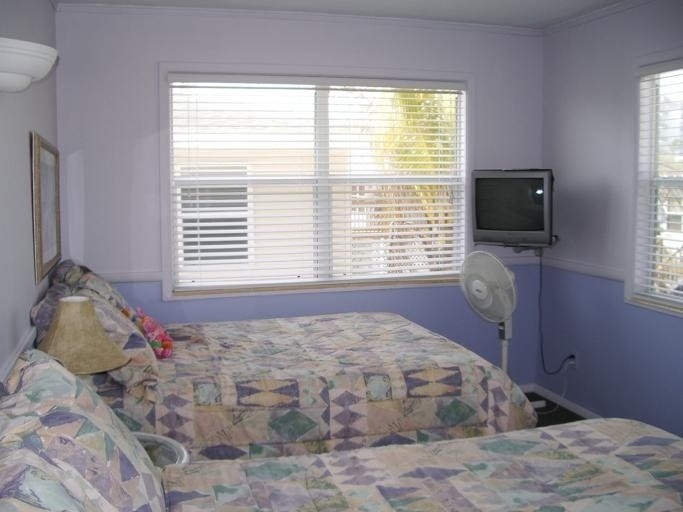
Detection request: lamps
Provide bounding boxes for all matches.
[36,296,131,394]
[0,37,58,94]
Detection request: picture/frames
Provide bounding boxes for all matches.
[28,130,62,285]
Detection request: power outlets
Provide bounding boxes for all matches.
[563,351,578,371]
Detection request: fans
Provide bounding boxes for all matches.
[460,251,518,377]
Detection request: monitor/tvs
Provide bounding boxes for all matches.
[471,169,553,248]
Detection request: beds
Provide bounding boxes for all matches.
[0,351,681,511]
[37,254,525,464]
